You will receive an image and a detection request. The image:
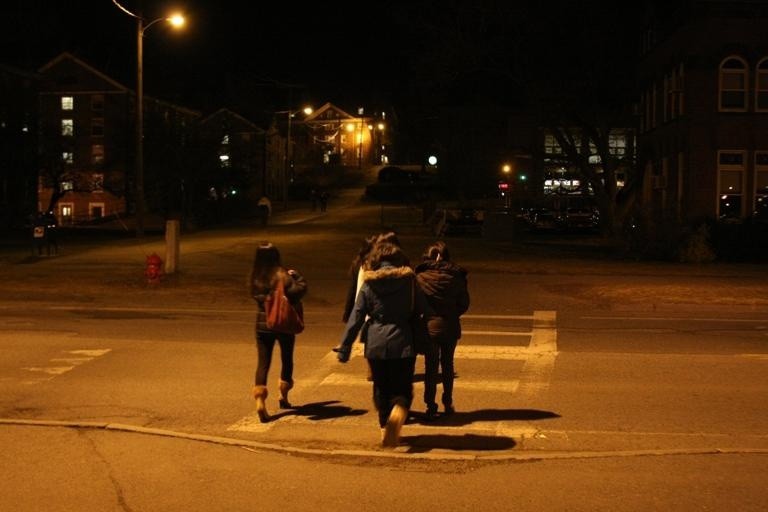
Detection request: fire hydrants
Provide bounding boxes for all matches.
[143,253,163,285]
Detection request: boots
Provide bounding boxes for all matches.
[253,385,270,423]
[278,381,291,409]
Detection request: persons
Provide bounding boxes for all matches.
[249,241,310,422]
[319,191,329,213]
[341,234,376,380]
[413,239,472,419]
[331,242,428,449]
[355,231,403,323]
[310,190,318,211]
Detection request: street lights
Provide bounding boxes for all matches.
[422,156,443,221]
[286,107,311,178]
[358,124,384,171]
[135,12,184,231]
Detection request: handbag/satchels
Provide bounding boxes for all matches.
[264,279,305,334]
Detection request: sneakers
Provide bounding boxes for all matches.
[426,403,454,418]
[381,404,407,446]
[367,366,374,381]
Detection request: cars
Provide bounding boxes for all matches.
[529,207,600,229]
